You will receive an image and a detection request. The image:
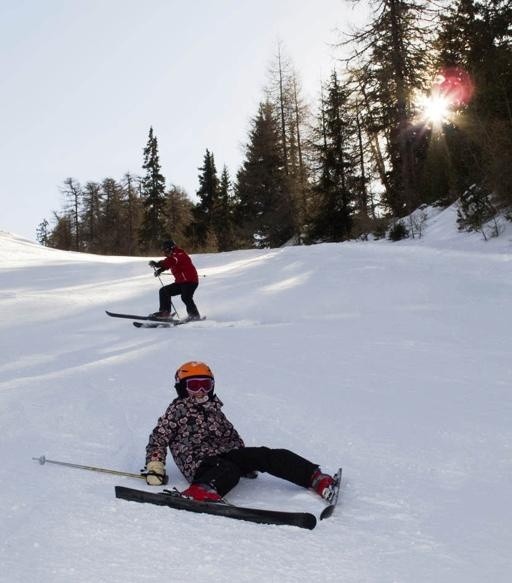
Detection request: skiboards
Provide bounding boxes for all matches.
[105,311,208,327]
[115,467,344,529]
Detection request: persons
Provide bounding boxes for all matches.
[140,359,336,503]
[149,239,201,319]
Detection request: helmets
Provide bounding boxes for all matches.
[161,238,176,252]
[174,361,214,385]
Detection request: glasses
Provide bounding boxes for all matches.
[185,378,215,394]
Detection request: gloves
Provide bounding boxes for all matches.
[154,269,162,276]
[149,260,162,268]
[144,461,165,487]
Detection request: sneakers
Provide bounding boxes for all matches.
[180,480,223,503]
[184,313,200,322]
[309,468,336,501]
[150,311,171,318]
